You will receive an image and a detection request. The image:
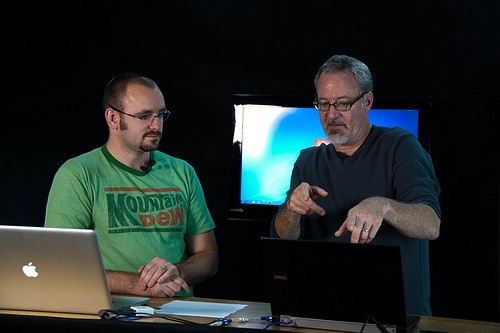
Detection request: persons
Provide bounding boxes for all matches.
[269,55,441,317]
[44,72,219,298]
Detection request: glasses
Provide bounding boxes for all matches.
[105,101,170,123]
[313,92,366,111]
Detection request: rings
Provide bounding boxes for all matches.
[363,228,370,232]
[161,265,167,271]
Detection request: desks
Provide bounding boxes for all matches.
[0,297,500,333]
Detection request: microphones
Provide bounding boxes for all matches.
[336,151,345,159]
[148,159,156,166]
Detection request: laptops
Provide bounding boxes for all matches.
[260,236,421,333]
[0,225,152,315]
